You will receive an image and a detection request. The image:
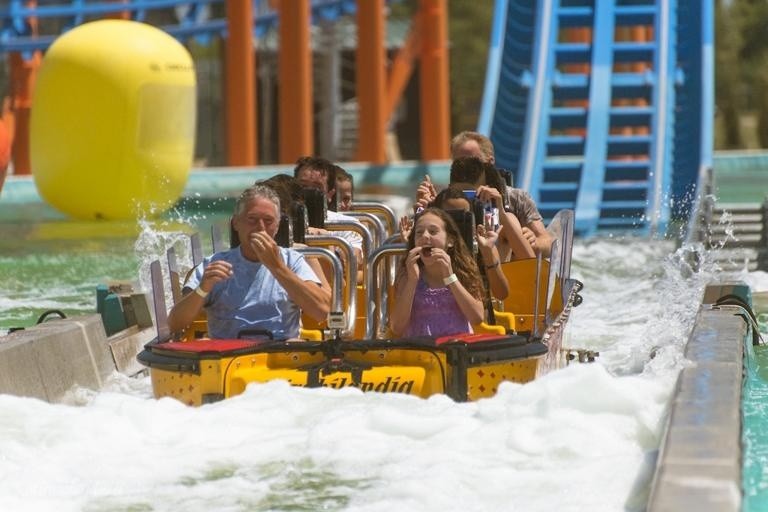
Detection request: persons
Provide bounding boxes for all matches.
[167,185,335,342]
[386,206,489,343]
[254,130,554,310]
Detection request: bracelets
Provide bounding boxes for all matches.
[193,283,210,298]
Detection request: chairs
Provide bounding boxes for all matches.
[181,202,417,342]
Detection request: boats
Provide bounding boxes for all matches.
[138,192,599,406]
